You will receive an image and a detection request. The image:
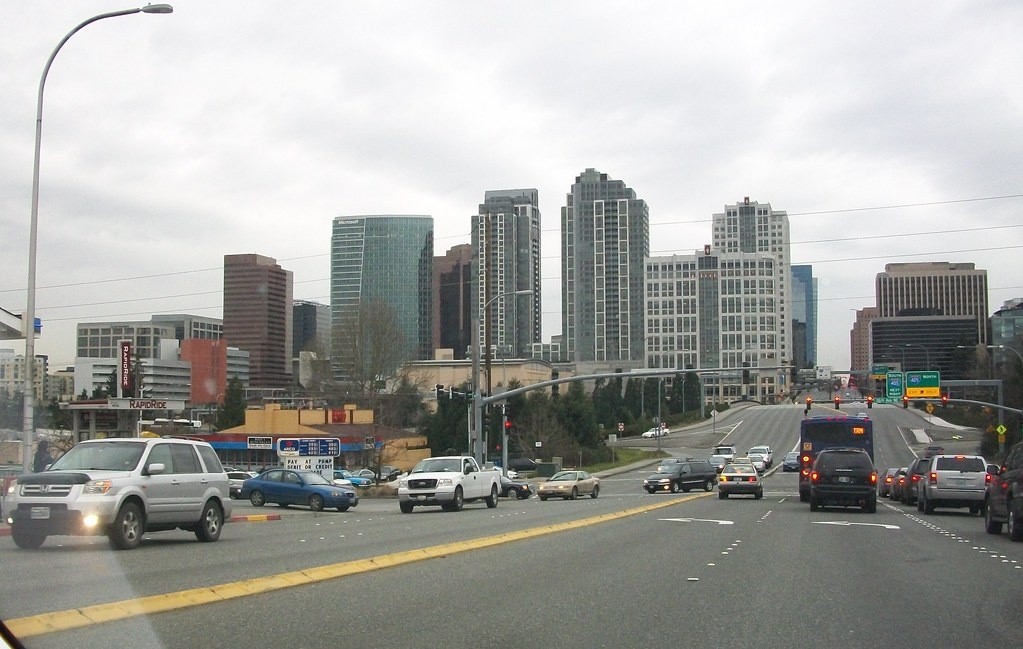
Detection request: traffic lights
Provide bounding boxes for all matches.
[505,403,511,415]
[435,384,486,406]
[483,413,492,432]
[505,421,511,435]
[805,396,909,410]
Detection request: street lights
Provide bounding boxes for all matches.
[888,342,993,393]
[22,1,173,472]
[474,289,535,469]
[491,347,507,474]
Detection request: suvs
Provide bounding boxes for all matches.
[3,435,234,549]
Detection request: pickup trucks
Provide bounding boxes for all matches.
[399,455,502,513]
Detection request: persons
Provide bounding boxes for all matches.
[33,439,56,473]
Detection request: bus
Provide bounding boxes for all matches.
[797,414,873,499]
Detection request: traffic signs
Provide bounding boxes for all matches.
[905,371,940,397]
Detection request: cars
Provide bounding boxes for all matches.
[536,470,600,500]
[498,476,536,500]
[493,457,538,478]
[241,468,359,514]
[222,466,260,499]
[641,427,1023,542]
[331,465,402,487]
